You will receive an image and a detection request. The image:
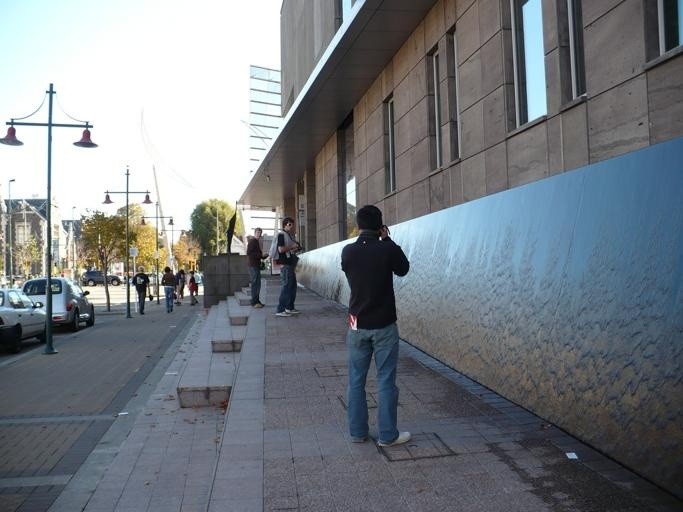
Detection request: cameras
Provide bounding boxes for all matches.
[297,246,303,252]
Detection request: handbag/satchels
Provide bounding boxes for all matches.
[148,295,153,301]
[173,292,177,299]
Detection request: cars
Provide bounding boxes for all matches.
[0,288,47,354]
[22,277,94,332]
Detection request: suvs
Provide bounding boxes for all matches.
[82,271,121,286]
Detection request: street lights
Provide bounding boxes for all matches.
[103,165,153,317]
[140,202,174,304]
[0,84,98,354]
[9,180,15,288]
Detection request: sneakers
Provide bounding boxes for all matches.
[290,309,301,314]
[376,432,410,447]
[253,303,262,308]
[275,311,293,317]
[258,300,264,306]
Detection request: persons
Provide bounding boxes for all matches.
[161,267,186,313]
[132,266,150,314]
[247,227,268,308]
[194,271,200,296]
[189,271,199,306]
[341,205,411,447]
[274,218,302,316]
